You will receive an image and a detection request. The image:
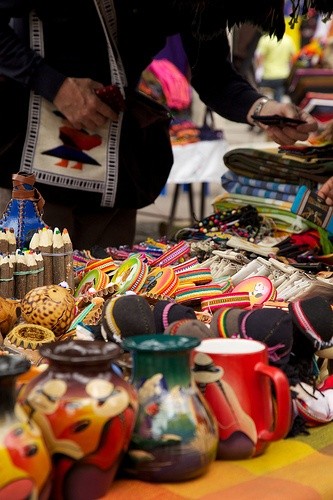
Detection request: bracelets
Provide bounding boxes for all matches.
[251,97,272,126]
[170,203,275,245]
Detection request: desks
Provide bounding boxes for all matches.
[163,140,228,228]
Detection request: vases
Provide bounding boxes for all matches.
[0,333,219,500]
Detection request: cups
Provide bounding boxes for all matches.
[191,337,291,461]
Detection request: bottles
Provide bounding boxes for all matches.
[18,339,139,500]
[118,334,217,484]
[0,354,53,500]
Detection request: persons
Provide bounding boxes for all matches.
[319,177,333,206]
[0,0,333,251]
